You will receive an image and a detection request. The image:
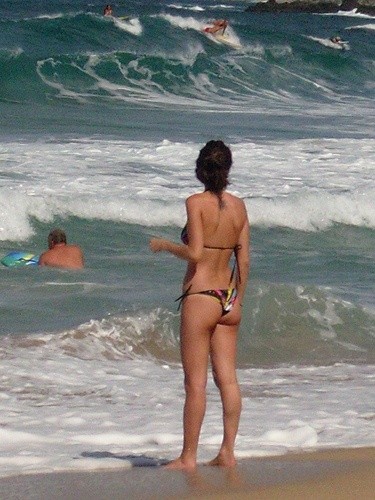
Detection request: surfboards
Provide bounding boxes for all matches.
[0,252,41,269]
[209,32,242,49]
[112,13,140,21]
[331,41,350,44]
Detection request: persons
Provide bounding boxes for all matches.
[104,5,113,15]
[201,18,230,36]
[36,229,85,269]
[332,37,348,43]
[149,137,254,469]
